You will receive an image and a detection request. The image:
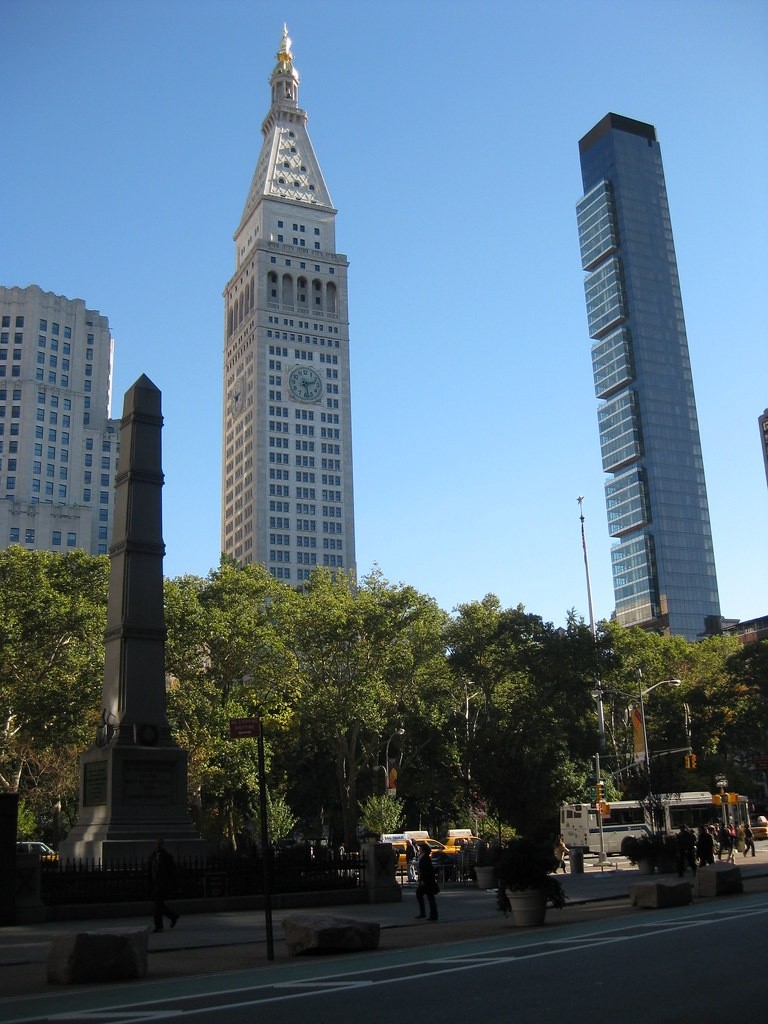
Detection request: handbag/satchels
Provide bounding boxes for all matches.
[432,881,440,895]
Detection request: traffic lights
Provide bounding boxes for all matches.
[721,793,731,803]
[596,784,605,801]
[684,755,691,769]
[591,802,596,808]
[691,754,697,769]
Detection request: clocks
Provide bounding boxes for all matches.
[286,365,326,406]
[230,377,248,418]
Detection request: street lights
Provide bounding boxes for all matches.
[372,728,405,796]
[590,667,682,835]
[464,680,484,776]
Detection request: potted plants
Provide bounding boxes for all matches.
[474,846,500,888]
[498,854,570,927]
[620,836,660,874]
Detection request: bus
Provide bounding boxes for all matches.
[559,792,752,857]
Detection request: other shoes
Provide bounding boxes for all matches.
[170,912,180,928]
[427,917,438,921]
[414,915,425,918]
[153,928,164,933]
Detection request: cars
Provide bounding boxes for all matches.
[16,841,58,862]
[440,829,491,852]
[379,829,461,872]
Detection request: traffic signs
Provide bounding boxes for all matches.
[713,780,729,788]
[713,774,727,780]
[230,716,262,739]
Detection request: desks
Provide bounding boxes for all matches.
[400,864,456,888]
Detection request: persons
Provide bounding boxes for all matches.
[405,840,416,883]
[552,834,570,874]
[414,844,439,921]
[146,838,181,934]
[674,816,756,880]
